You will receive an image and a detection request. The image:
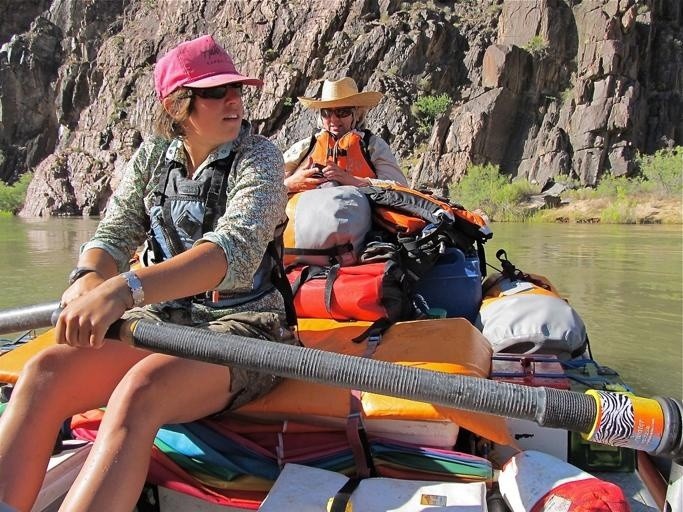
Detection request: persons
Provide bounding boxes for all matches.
[1,34,305,512]
[279,76,412,205]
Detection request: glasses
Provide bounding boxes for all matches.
[321,109,352,118]
[178,82,243,99]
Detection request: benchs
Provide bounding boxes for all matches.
[0,317,483,444]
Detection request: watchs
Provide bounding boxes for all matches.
[66,262,108,285]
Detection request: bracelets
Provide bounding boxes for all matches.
[118,266,149,309]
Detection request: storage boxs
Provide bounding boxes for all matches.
[489,354,568,466]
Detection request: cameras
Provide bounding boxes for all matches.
[310,163,327,178]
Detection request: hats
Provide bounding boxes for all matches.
[296,77,383,108]
[154,34,264,104]
